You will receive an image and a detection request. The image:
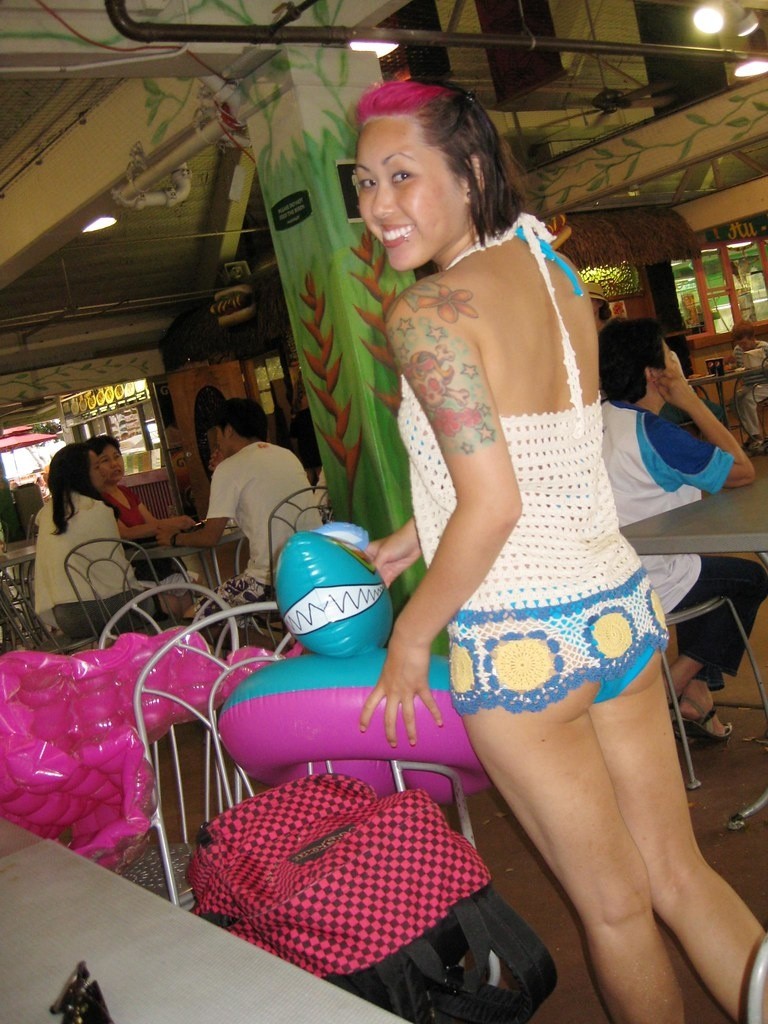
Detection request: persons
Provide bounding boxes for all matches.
[84,435,196,613]
[154,398,324,654]
[32,443,178,636]
[597,316,768,742]
[724,320,768,450]
[586,282,615,331]
[352,80,768,1024]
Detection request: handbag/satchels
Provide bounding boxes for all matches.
[742,348,768,370]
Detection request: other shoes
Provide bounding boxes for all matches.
[742,435,763,452]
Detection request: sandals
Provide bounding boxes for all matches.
[661,671,684,710]
[672,695,733,743]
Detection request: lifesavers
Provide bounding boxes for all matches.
[214,519,497,804]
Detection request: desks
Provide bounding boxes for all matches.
[620,478,768,829]
[687,364,768,432]
[125,523,247,643]
[0,536,37,650]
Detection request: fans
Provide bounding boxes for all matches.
[539,0,675,131]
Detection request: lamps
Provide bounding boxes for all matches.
[694,0,759,39]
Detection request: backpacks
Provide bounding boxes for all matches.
[185,772,559,1024]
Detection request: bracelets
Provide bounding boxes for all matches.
[170,533,177,547]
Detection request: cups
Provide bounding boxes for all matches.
[705,358,717,377]
[713,356,725,377]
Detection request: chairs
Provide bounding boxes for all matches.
[96,583,238,906]
[0,567,96,654]
[64,537,214,645]
[133,603,505,990]
[734,347,768,448]
[240,485,334,652]
[660,593,768,790]
